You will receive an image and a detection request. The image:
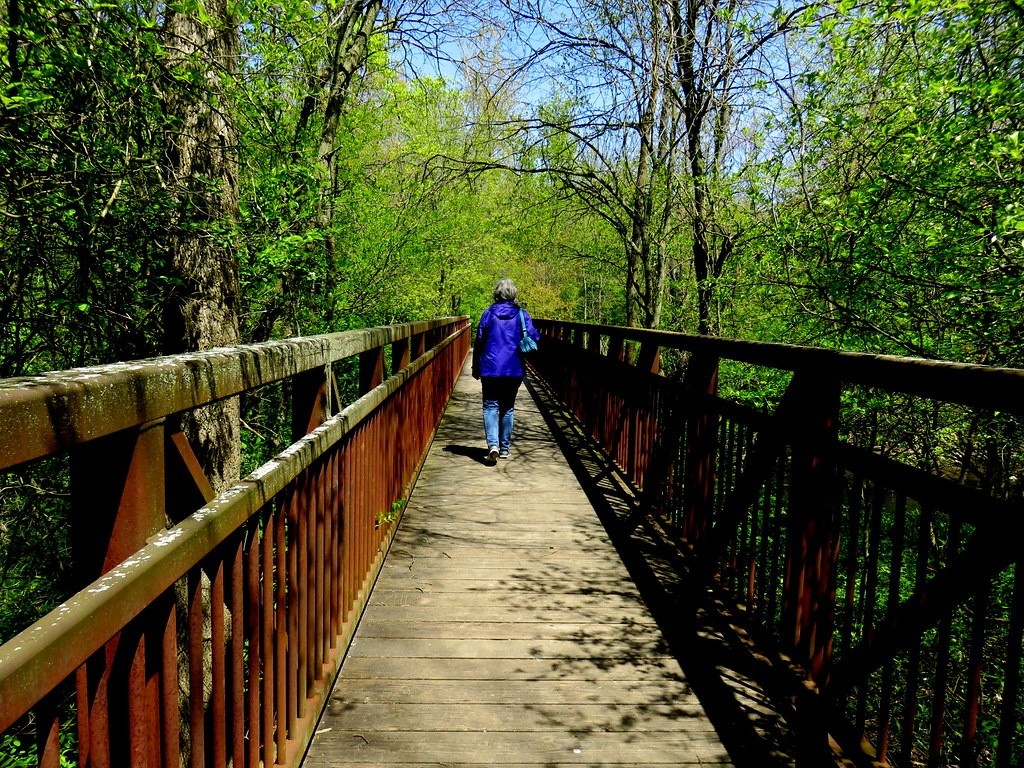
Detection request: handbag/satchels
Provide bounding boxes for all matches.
[519,308,538,353]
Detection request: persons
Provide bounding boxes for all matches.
[471,279,539,460]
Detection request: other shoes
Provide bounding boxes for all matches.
[486,445,499,466]
[499,450,512,458]
